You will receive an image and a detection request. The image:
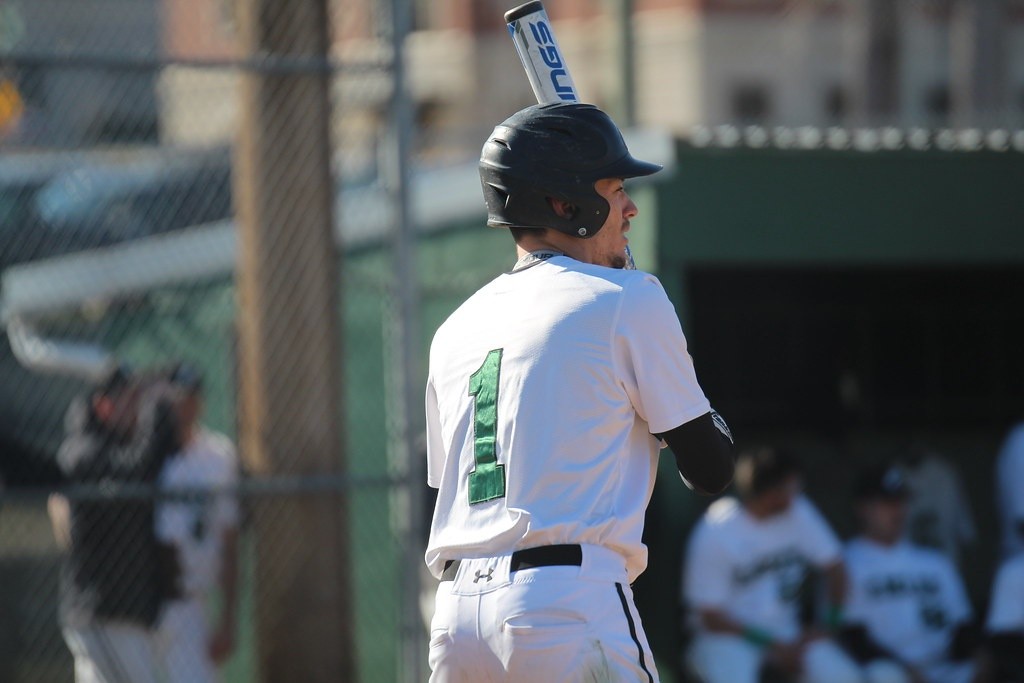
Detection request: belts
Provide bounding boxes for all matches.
[439,544,582,583]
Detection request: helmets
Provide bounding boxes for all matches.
[735,443,802,496]
[477,99,664,240]
[848,464,916,511]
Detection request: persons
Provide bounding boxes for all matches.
[678,426,1024,683]
[51,358,239,683]
[426,103,735,683]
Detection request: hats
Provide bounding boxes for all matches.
[81,358,164,396]
[154,357,207,392]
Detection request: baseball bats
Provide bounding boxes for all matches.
[504,1,637,270]
